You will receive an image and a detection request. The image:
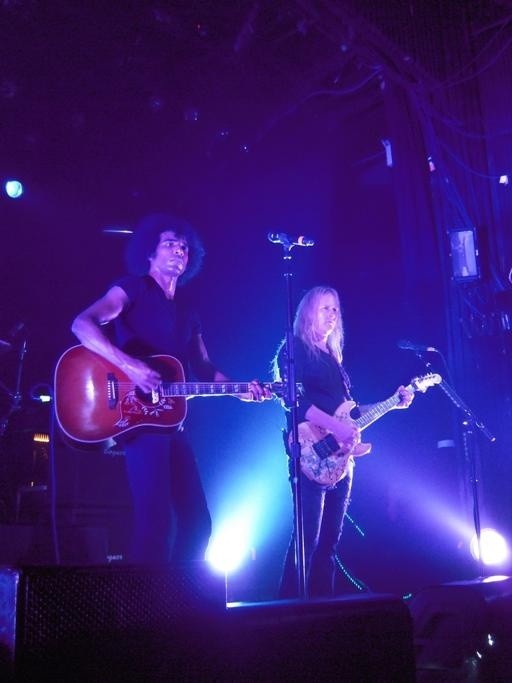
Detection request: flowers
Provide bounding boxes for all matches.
[267,232,315,248]
[395,338,438,352]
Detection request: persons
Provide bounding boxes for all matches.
[68,209,274,584]
[270,281,415,601]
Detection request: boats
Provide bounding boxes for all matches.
[223,591,420,682]
[14,562,223,683]
